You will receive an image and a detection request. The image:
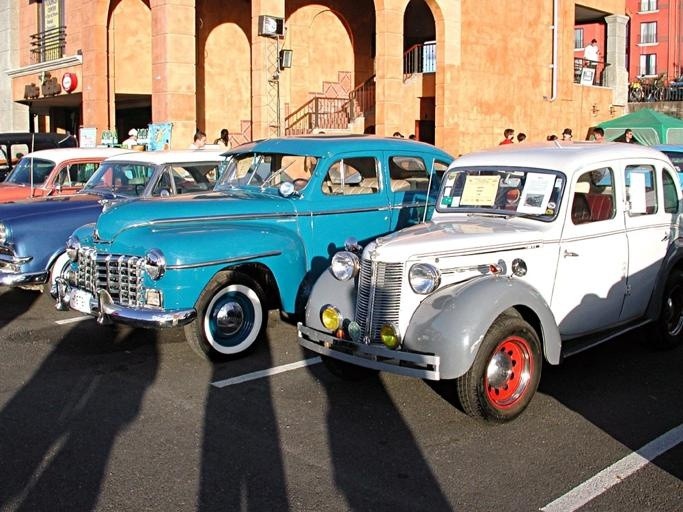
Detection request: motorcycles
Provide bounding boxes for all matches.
[627,74,668,102]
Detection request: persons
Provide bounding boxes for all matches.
[214,128,232,150]
[122,128,138,149]
[393,132,416,140]
[547,135,558,141]
[630,70,683,100]
[584,39,601,84]
[517,133,526,143]
[593,128,604,141]
[561,129,572,141]
[189,132,206,149]
[500,129,514,145]
[619,129,636,144]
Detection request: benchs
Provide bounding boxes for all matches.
[360,177,410,193]
[505,189,613,224]
[320,183,376,195]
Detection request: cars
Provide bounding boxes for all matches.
[0,146,141,207]
[0,149,241,303]
[292,146,681,426]
[600,144,682,206]
[57,135,454,364]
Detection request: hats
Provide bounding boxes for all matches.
[562,129,572,138]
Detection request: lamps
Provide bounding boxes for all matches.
[280,50,293,68]
